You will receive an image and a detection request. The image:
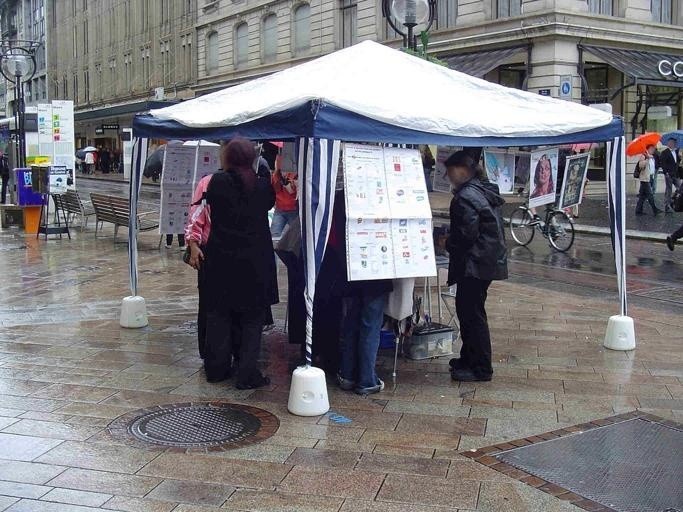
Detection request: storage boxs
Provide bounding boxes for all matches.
[376,328,398,350]
[403,319,453,361]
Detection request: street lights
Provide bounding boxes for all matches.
[381,0,436,49]
[0,39,43,166]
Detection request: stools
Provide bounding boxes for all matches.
[438,290,464,343]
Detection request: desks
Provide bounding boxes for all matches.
[282,243,461,380]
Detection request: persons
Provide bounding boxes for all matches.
[185,138,393,395]
[0,147,9,204]
[420,145,435,192]
[165,234,188,251]
[442,152,508,382]
[530,154,555,198]
[636,138,683,251]
[76,145,110,175]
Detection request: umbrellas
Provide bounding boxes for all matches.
[143,139,184,179]
[659,129,683,149]
[625,132,662,157]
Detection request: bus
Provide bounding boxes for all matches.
[0,106,38,163]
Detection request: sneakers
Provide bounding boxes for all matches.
[448,357,494,372]
[336,370,357,390]
[165,243,172,249]
[357,377,386,395]
[236,375,271,389]
[667,236,675,252]
[179,245,187,251]
[205,368,233,383]
[451,369,492,382]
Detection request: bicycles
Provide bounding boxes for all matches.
[509,187,575,252]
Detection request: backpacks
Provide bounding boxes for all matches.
[670,184,683,213]
[633,153,646,178]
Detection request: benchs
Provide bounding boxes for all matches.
[88,190,162,248]
[48,185,104,233]
